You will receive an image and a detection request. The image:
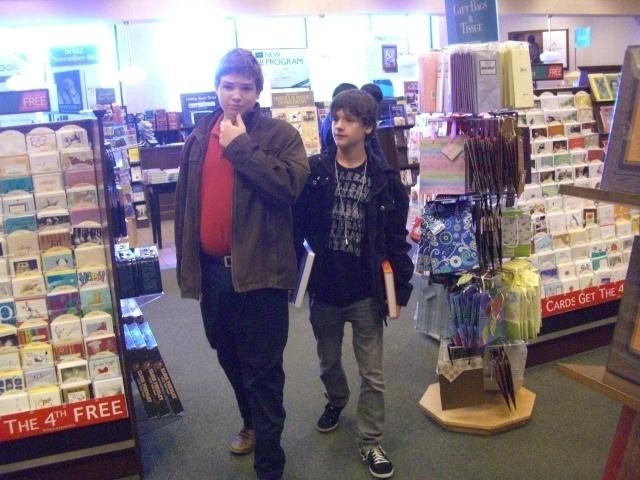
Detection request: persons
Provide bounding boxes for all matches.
[291,89,414,479]
[319,82,383,154]
[174,46,311,480]
[527,35,543,64]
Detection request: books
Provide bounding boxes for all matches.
[289,237,316,307]
[380,258,398,319]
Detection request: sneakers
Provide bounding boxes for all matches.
[315,400,348,433]
[358,438,394,479]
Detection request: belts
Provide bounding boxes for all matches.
[200,255,232,267]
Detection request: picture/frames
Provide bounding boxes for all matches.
[597,44,639,387]
[382,45,398,71]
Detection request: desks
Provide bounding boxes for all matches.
[143,180,176,251]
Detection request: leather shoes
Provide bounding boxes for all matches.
[228,425,256,454]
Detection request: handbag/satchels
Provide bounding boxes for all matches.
[415,118,542,344]
[438,345,487,411]
